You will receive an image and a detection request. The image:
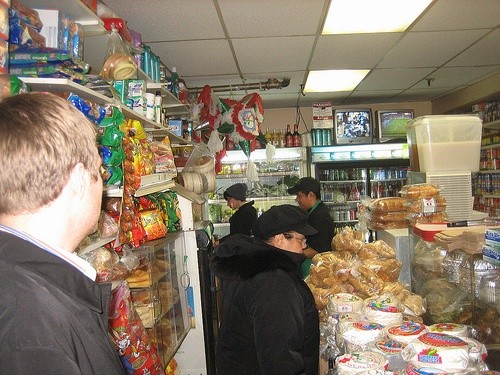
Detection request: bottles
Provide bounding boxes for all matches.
[141,45,160,83]
[172,67,179,99]
[278,128,286,147]
[271,128,279,148]
[292,124,301,146]
[286,125,293,147]
[481,133,500,146]
[264,127,271,145]
[155,91,162,124]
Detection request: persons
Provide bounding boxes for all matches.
[214,183,258,247]
[211,204,321,375]
[285,177,333,280]
[0,91,126,375]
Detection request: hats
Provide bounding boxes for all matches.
[256,205,318,240]
[287,176,320,195]
[226,183,248,200]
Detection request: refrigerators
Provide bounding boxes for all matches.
[310,143,409,234]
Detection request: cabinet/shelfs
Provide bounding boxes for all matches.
[208,146,308,203]
[120,230,192,371]
[0,0,192,257]
[472,119,500,220]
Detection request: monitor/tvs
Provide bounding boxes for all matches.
[333,108,374,145]
[376,109,414,143]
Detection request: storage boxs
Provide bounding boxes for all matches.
[34,10,84,61]
[114,79,146,115]
